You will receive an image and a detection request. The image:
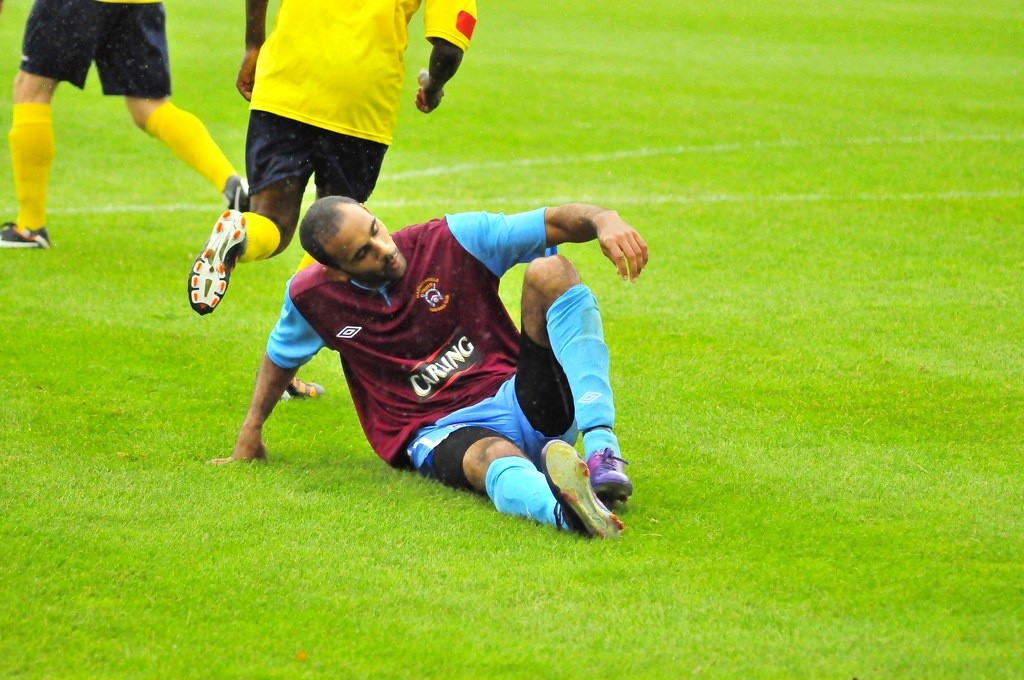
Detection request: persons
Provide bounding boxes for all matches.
[186,0,477,399]
[2,0,248,247]
[208,197,649,537]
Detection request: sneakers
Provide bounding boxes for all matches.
[586,448,632,498]
[187,210,247,315]
[0,222,50,247]
[229,178,249,212]
[282,376,325,401]
[541,440,625,538]
